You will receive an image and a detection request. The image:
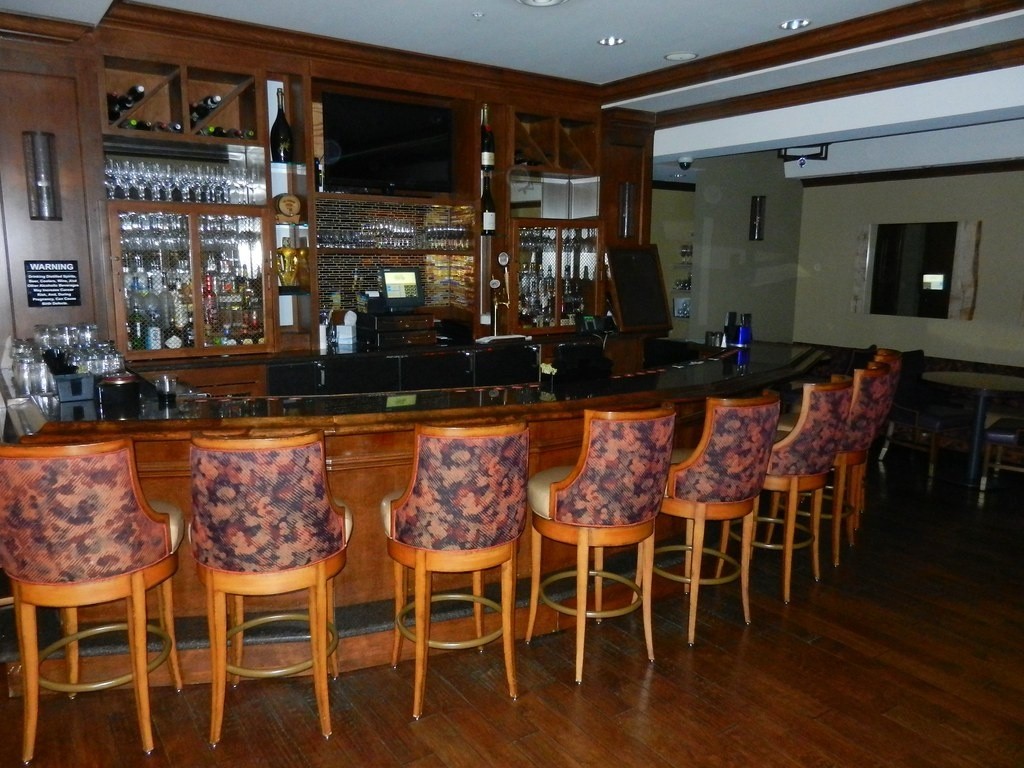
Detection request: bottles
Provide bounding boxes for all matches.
[201,254,266,347]
[117,120,183,134]
[705,332,722,347]
[270,88,294,163]
[122,254,196,351]
[100,375,142,420]
[178,398,307,419]
[481,170,496,236]
[190,96,222,130]
[196,126,256,139]
[561,265,592,319]
[107,86,145,125]
[481,103,495,169]
[519,263,555,327]
[514,148,581,169]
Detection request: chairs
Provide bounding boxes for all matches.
[980,417,1024,492]
[877,349,981,486]
[381,424,530,716]
[524,345,901,683]
[188,428,355,744]
[0,437,185,765]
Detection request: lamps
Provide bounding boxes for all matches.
[750,194,767,242]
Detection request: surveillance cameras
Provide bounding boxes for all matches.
[677,157,694,170]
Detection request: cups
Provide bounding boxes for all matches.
[54,341,124,374]
[11,338,61,421]
[152,375,177,411]
[723,326,751,345]
[34,324,99,344]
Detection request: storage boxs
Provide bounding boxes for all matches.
[55,371,96,403]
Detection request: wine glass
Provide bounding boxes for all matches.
[360,217,417,250]
[104,158,257,206]
[117,212,263,253]
[678,303,690,318]
[519,228,598,252]
[316,231,374,249]
[424,226,471,251]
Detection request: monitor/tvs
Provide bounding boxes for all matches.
[381,267,424,306]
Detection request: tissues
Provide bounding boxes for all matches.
[335,311,358,345]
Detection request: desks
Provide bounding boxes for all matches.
[920,371,1023,490]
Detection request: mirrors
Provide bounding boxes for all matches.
[848,220,983,322]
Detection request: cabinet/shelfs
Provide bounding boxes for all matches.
[669,262,691,322]
[86,25,657,397]
